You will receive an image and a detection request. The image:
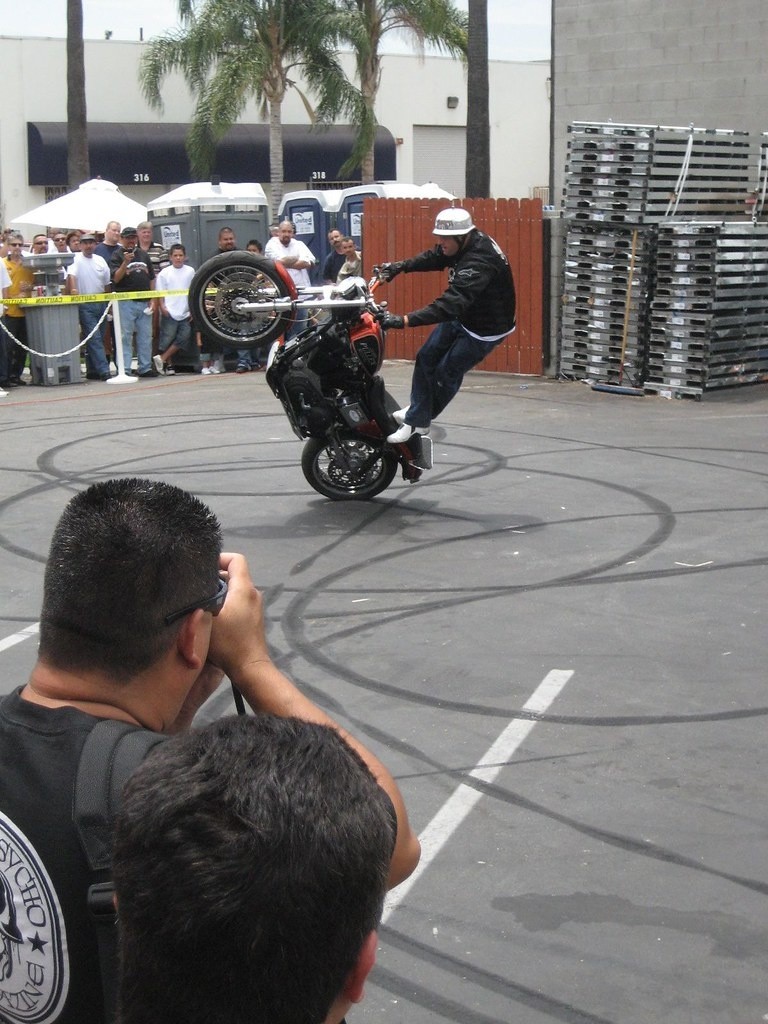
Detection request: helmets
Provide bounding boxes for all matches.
[432,207,476,236]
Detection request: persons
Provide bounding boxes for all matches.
[0,477,421,1024]
[373,206,516,443]
[0,221,361,395]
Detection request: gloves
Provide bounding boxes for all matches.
[372,311,404,332]
[381,260,406,282]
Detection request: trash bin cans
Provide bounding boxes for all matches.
[18,253,82,387]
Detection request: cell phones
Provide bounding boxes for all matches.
[127,248,133,253]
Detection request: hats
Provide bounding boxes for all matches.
[79,233,96,243]
[121,227,138,238]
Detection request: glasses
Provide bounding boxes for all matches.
[35,241,48,245]
[8,243,23,247]
[53,238,66,241]
[168,575,227,627]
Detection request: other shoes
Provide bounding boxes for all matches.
[140,370,159,377]
[101,372,111,380]
[86,373,102,380]
[2,377,26,387]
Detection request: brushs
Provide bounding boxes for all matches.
[591,228,646,397]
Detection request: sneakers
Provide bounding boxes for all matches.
[235,358,262,372]
[166,364,175,375]
[201,366,220,376]
[152,354,165,376]
[386,423,431,443]
[393,404,411,425]
[215,361,226,373]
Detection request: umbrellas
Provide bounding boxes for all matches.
[10,175,148,240]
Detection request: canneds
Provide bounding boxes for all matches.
[38,286,43,296]
[55,288,62,295]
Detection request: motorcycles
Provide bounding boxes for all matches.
[188,250,448,502]
